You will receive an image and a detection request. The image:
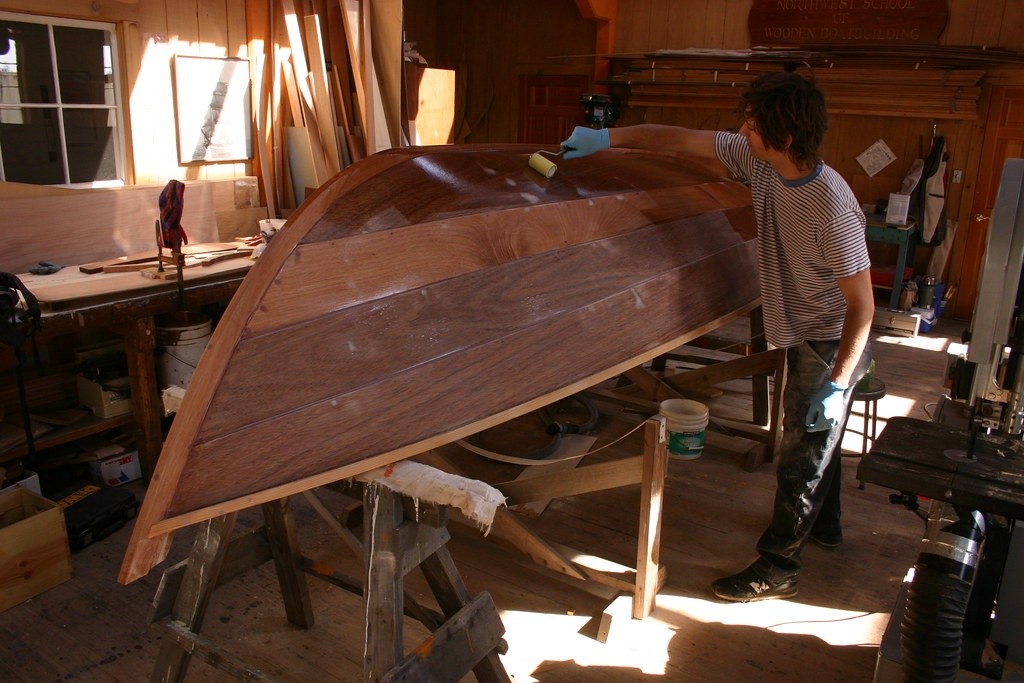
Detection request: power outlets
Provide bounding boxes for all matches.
[952,169,962,184]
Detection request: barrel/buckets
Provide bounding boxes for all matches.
[153,310,214,389]
[659,398,709,460]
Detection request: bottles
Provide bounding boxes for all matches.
[858,358,876,393]
[919,276,936,310]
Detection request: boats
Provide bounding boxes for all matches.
[116,143,761,585]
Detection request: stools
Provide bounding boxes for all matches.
[841,377,886,491]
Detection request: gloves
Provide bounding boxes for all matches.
[560,127,610,159]
[805,379,848,433]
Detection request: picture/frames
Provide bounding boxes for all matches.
[174,50,255,167]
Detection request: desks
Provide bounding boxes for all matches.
[864,216,917,310]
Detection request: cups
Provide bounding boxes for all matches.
[258,219,288,244]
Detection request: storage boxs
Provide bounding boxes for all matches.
[870,263,914,288]
[40,470,138,553]
[872,306,922,338]
[89,447,143,489]
[73,339,135,419]
[0,469,43,496]
[0,487,74,614]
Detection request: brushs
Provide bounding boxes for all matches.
[528,147,575,179]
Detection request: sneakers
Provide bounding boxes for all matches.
[711,564,799,602]
[807,525,843,548]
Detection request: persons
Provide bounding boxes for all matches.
[558,70,876,600]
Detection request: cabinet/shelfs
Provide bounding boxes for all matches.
[0,240,256,487]
